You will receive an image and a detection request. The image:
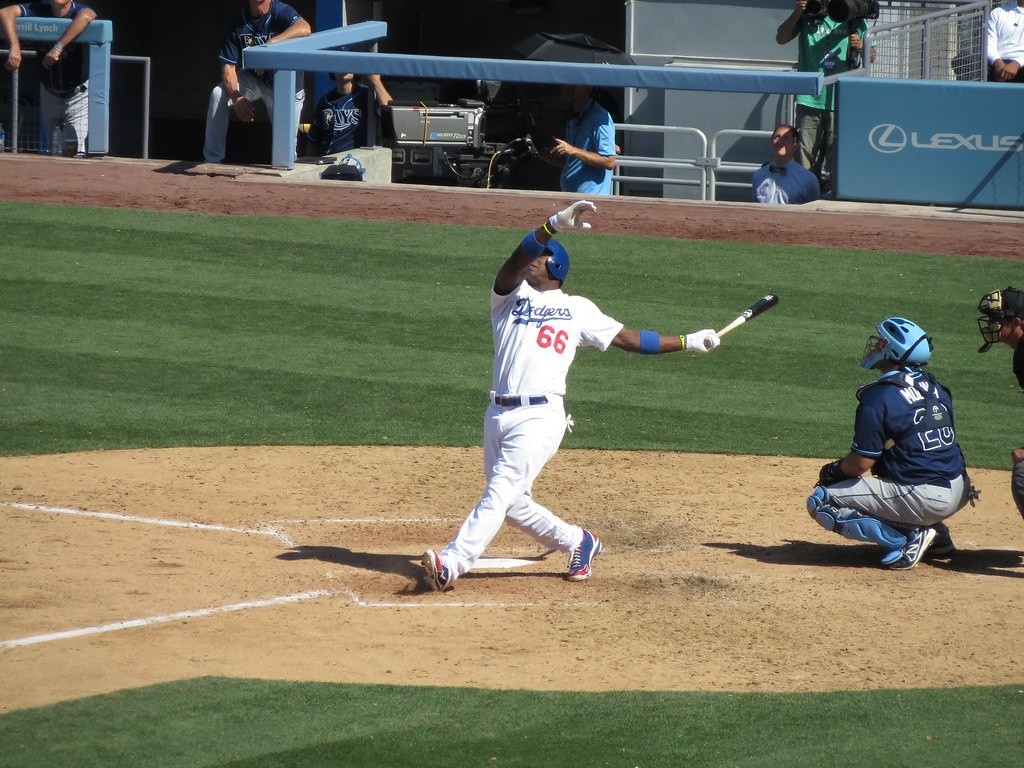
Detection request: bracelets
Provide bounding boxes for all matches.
[53,45,63,53]
[679,334,686,350]
[542,222,553,238]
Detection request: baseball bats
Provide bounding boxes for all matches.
[704,293,780,347]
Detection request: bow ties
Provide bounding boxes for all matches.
[769,164,787,176]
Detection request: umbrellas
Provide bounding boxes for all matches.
[506,32,637,66]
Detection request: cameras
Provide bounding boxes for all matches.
[803,0,825,13]
[828,0,879,22]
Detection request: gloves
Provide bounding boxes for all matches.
[686,329,720,352]
[820,458,858,487]
[549,200,597,232]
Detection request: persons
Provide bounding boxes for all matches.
[421,200,721,592]
[750,124,820,206]
[777,0,877,198]
[301,45,395,159]
[805,316,973,570]
[549,85,616,196]
[986,0,1024,84]
[977,287,1024,522]
[0,0,97,157]
[202,0,312,164]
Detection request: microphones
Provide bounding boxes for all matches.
[386,99,458,107]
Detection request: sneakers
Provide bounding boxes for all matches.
[928,522,954,555]
[423,549,448,591]
[564,529,602,582]
[889,526,936,570]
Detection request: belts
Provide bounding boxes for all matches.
[45,86,87,100]
[495,396,548,405]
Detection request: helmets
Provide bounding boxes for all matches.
[977,286,1024,352]
[861,317,931,369]
[545,239,570,288]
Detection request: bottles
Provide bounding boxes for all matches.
[0,123,6,153]
[50,126,63,156]
